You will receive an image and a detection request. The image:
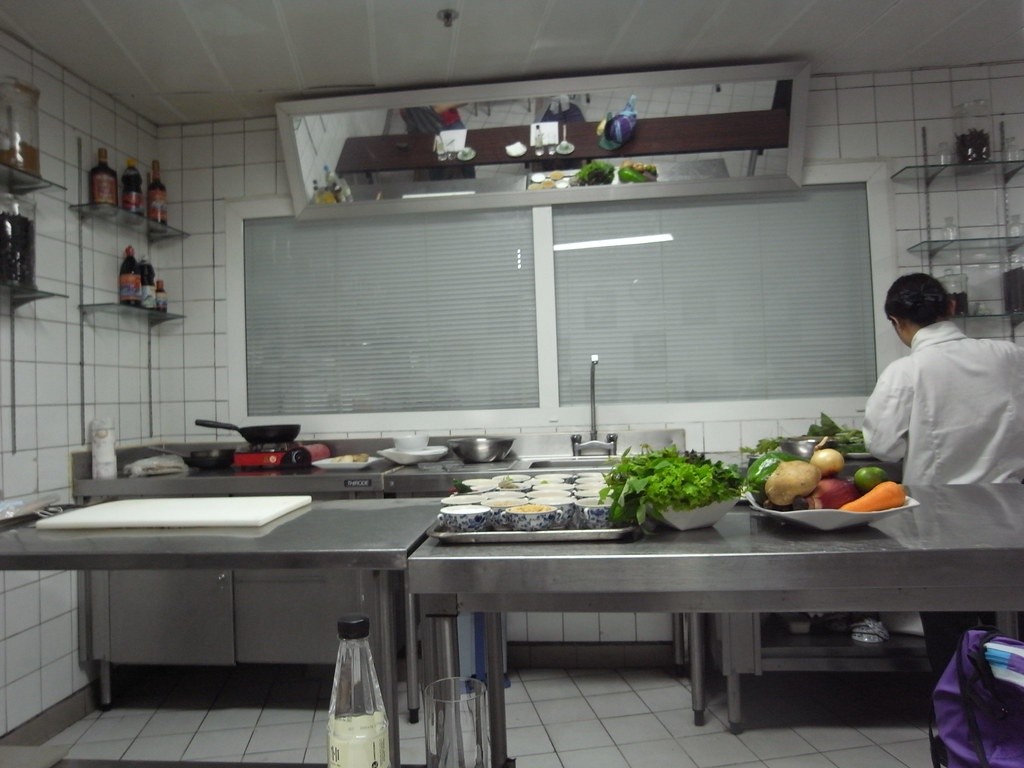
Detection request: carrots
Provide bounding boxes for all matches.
[840,481,905,512]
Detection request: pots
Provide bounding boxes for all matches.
[196,420,301,443]
[148,446,235,469]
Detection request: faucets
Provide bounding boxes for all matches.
[590,354,599,441]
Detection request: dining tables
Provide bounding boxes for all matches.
[335,108,790,190]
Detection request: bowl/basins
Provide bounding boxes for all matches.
[437,472,613,533]
[462,147,471,155]
[528,171,569,189]
[448,436,516,462]
[560,142,569,149]
[779,437,838,458]
[645,496,740,531]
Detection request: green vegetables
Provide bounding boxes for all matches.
[576,161,614,180]
[740,411,865,455]
[599,444,745,526]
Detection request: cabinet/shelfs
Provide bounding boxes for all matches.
[69,203,192,327]
[890,121,1023,319]
[0,162,70,309]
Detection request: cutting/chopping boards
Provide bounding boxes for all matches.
[35,496,313,530]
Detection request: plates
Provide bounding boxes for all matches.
[556,143,575,154]
[745,489,920,530]
[457,150,476,161]
[311,456,378,469]
[534,125,545,156]
[846,453,872,459]
[377,446,449,464]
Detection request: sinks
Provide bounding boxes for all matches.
[528,461,615,468]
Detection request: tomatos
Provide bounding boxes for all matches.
[855,466,889,494]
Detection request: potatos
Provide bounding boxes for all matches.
[765,460,822,505]
[619,160,644,173]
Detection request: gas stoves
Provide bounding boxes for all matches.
[233,443,330,468]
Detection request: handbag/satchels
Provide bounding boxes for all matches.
[596,95,638,151]
[928,624,1024,768]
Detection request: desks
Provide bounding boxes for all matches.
[406,481,1024,768]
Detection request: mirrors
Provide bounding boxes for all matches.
[273,56,814,222]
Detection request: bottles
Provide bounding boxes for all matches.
[313,165,354,204]
[937,142,951,164]
[955,97,990,161]
[436,135,447,161]
[0,192,38,290]
[328,616,391,768]
[119,245,157,310]
[943,216,959,240]
[0,75,40,177]
[121,159,144,226]
[1005,137,1019,160]
[88,148,118,217]
[936,269,968,315]
[1002,255,1024,314]
[147,160,167,232]
[155,279,167,313]
[1008,215,1024,236]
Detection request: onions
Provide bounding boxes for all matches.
[810,449,844,475]
[810,478,857,510]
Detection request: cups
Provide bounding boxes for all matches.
[424,677,492,768]
[446,143,456,160]
[548,138,556,154]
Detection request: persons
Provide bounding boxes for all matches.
[862,272,1024,675]
